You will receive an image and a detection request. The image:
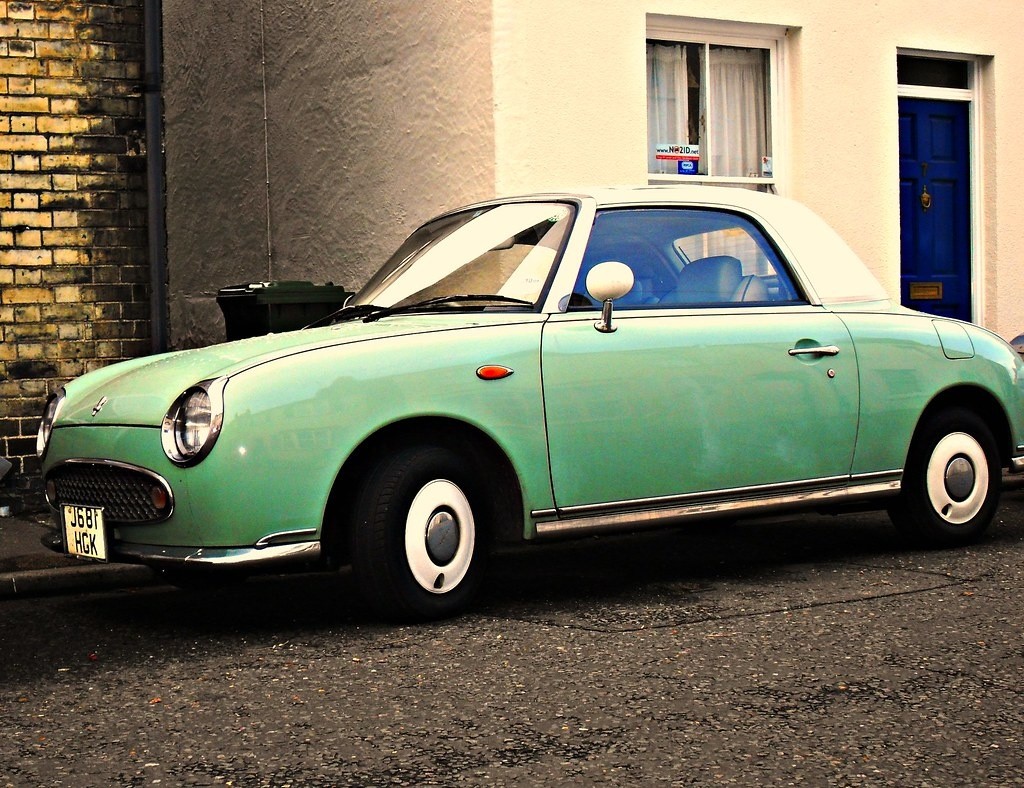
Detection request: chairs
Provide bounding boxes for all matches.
[615,277,660,305]
[658,256,770,306]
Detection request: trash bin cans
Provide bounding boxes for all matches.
[214,278,358,342]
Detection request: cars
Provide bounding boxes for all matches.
[35,183,1024,625]
